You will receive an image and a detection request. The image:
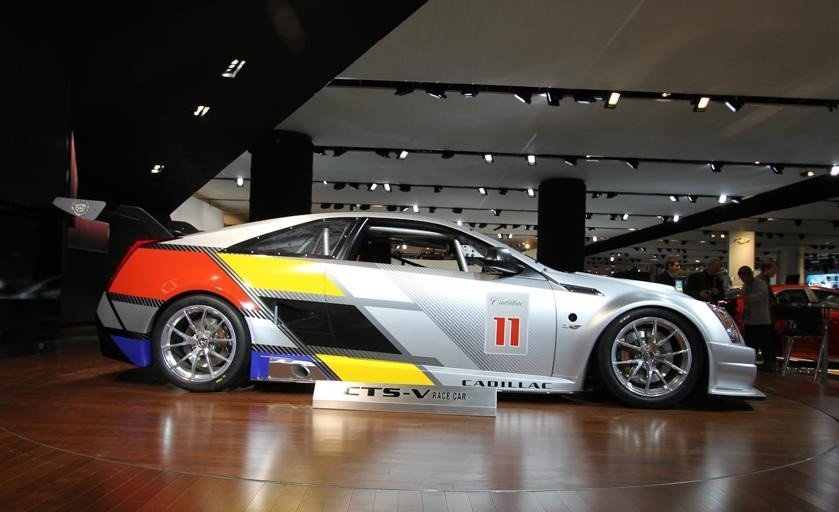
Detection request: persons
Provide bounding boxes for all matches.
[683,257,726,305]
[755,262,777,285]
[738,267,772,365]
[657,256,681,286]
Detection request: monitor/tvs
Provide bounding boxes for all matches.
[805,272,839,289]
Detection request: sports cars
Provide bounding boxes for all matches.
[51,196,767,408]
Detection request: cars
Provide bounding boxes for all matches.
[719,283,839,369]
